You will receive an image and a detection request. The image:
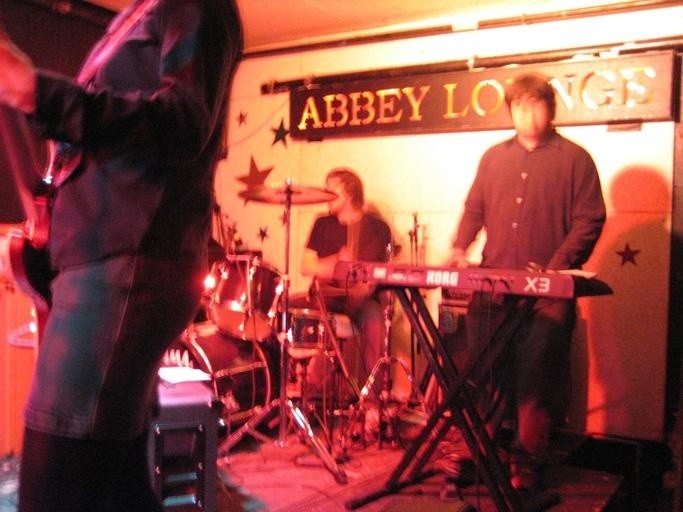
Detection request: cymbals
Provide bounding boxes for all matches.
[237,186,338,205]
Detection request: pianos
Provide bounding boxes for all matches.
[331,260,614,300]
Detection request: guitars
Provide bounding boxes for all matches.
[0,106,52,322]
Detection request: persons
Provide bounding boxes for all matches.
[432,76,607,497]
[301,169,400,402]
[0,1,243,510]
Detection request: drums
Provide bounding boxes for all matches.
[156,322,271,428]
[179,249,286,342]
[272,306,334,347]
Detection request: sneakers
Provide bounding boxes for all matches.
[433,454,542,493]
